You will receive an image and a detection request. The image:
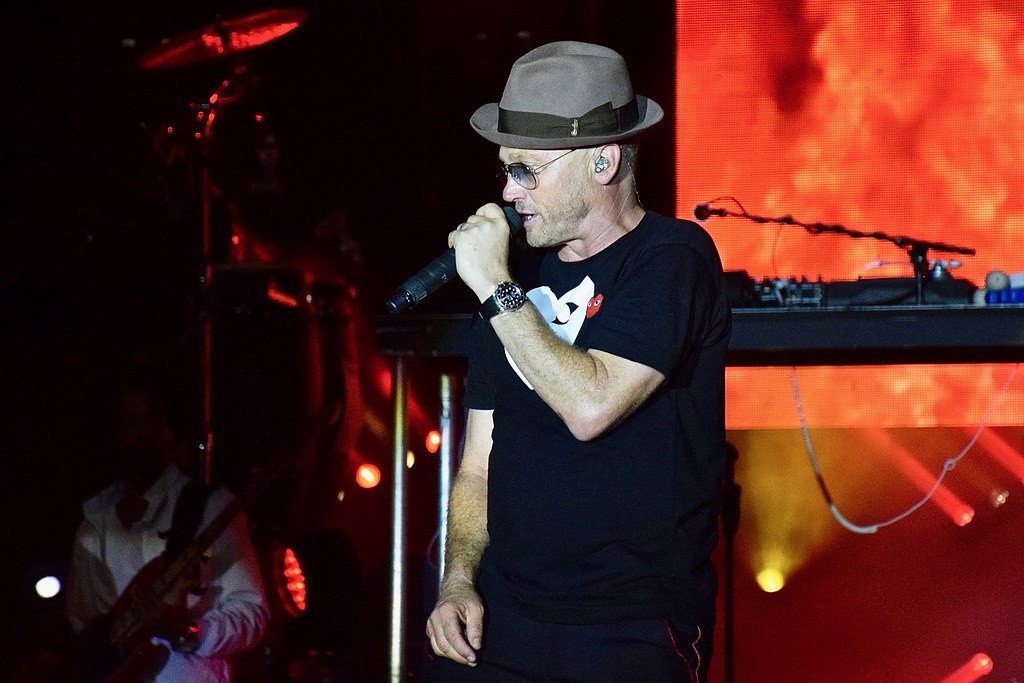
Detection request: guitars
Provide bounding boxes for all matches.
[75,448,288,677]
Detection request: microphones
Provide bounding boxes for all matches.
[385,205,523,315]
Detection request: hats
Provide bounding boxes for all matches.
[87,365,184,451]
[469,40,664,149]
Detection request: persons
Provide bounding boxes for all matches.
[66,382,269,682]
[426,41,729,682]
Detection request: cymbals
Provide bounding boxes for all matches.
[140,9,307,71]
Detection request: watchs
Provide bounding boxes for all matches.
[170,619,201,654]
[479,280,530,322]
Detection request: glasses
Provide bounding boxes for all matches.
[489,150,579,190]
